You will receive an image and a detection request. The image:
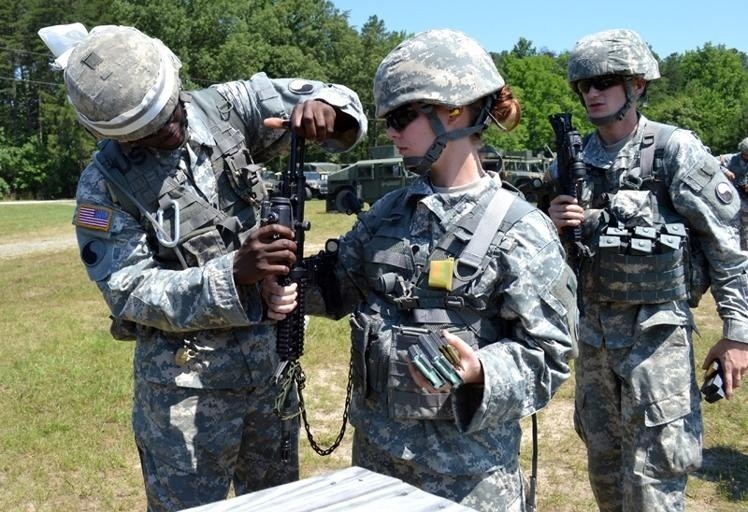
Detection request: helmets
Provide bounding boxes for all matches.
[63,23,184,145]
[565,27,662,90]
[737,137,748,155]
[368,26,507,121]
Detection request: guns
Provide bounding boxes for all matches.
[260,121,343,464]
[540,113,595,319]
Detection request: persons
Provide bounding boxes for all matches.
[56,24,369,511]
[713,138,748,252]
[261,27,581,512]
[536,29,748,511]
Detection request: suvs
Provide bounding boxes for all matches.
[257,145,550,211]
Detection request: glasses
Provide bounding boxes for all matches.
[578,74,636,95]
[386,103,423,133]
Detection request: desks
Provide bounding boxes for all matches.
[172,464,485,512]
